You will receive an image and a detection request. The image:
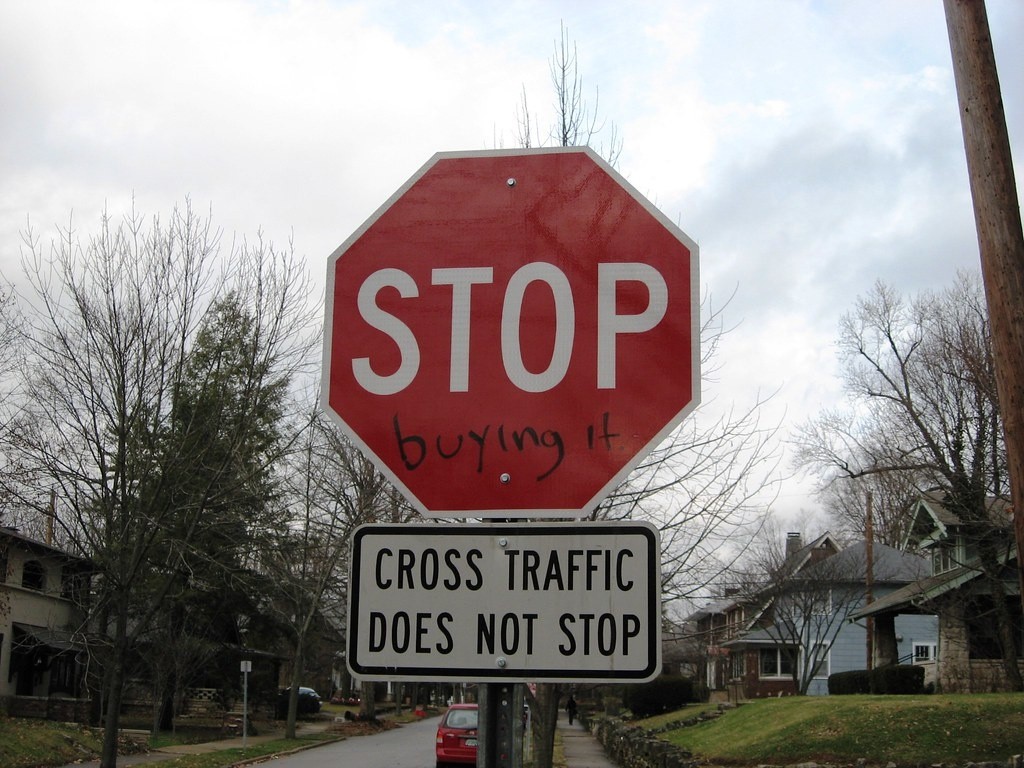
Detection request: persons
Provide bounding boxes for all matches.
[566,695,577,725]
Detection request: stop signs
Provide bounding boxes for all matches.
[318,146,703,519]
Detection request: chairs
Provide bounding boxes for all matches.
[456,717,467,726]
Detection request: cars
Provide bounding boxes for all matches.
[282,686,322,713]
[435,703,480,768]
